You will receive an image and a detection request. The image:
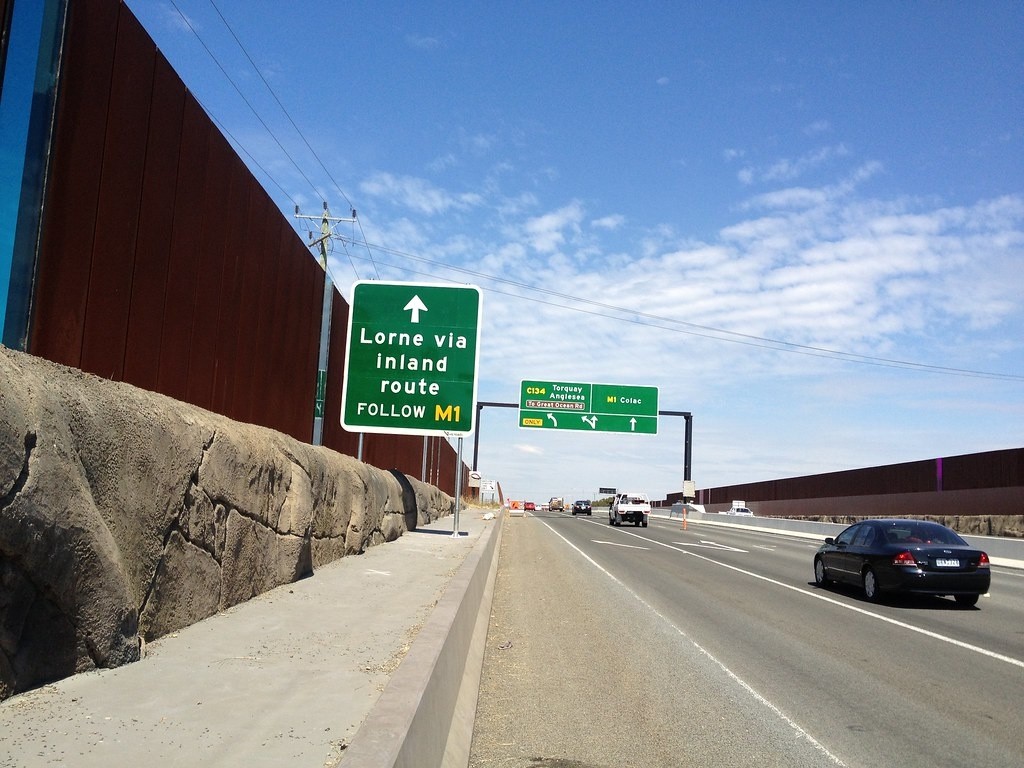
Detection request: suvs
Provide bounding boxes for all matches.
[525,502,535,511]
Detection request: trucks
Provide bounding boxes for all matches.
[549,497,563,511]
[608,493,651,527]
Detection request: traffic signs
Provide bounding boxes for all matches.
[519,379,658,435]
[341,280,480,440]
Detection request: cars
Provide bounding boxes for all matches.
[572,501,591,515]
[726,507,753,518]
[813,519,991,608]
